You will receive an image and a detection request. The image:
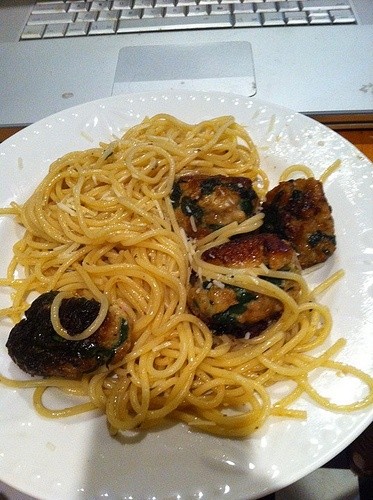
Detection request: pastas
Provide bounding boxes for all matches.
[0,112,372,437]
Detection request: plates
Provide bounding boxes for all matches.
[0,91,373,500]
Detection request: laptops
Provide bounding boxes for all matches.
[0,0,373,129]
